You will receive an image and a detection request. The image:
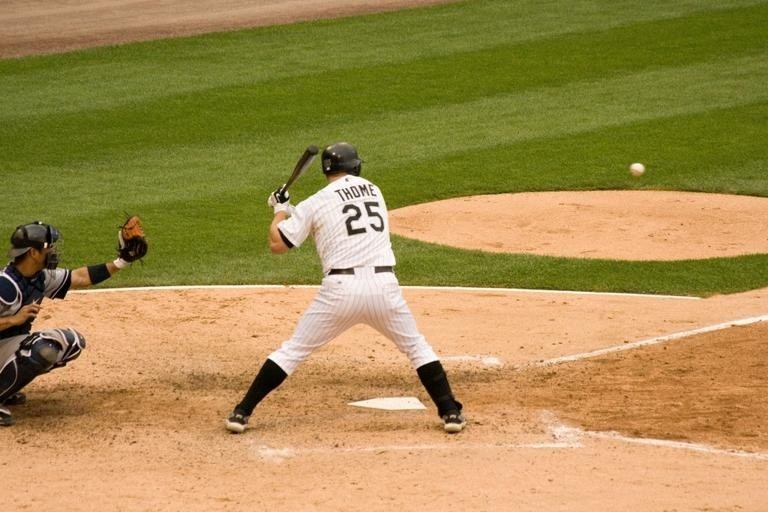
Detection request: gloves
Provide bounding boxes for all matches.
[268,184,290,213]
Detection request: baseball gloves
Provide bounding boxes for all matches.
[116,211,147,261]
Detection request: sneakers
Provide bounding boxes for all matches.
[226,409,248,432]
[442,411,467,432]
[0,393,25,426]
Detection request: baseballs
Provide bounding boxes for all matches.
[630,163,644,176]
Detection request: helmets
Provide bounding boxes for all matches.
[322,142,365,173]
[7,221,64,280]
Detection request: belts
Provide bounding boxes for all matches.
[328,266,393,275]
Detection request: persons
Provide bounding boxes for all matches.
[223,141,468,434]
[0,221,148,426]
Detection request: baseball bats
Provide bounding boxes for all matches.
[282,146,319,194]
